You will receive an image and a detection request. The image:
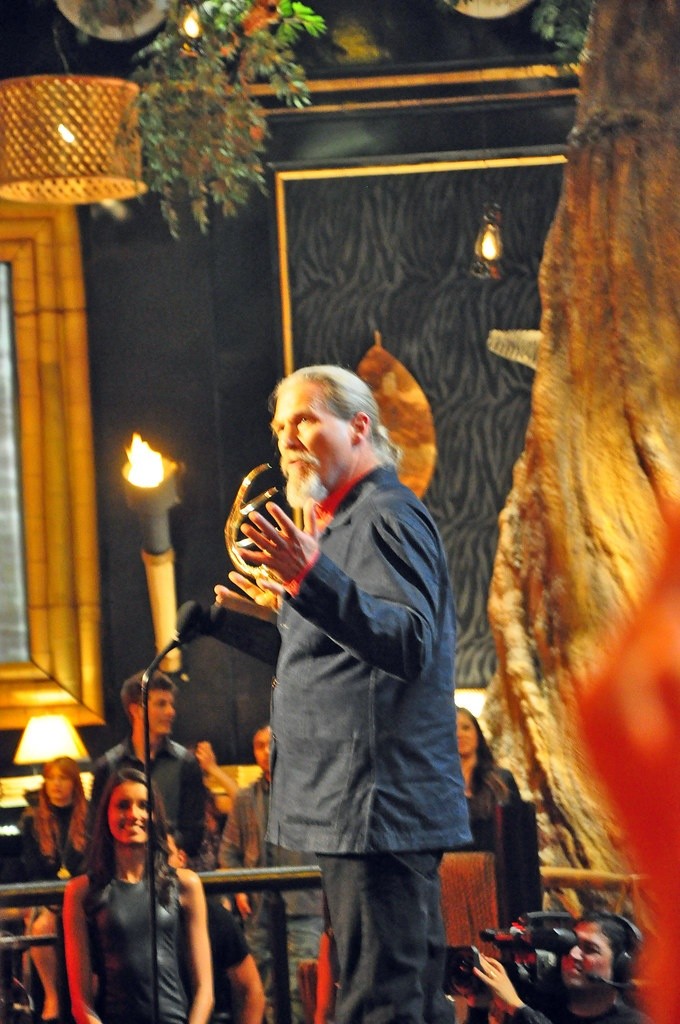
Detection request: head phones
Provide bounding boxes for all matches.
[611,914,641,991]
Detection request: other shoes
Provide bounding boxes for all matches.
[39,1016,60,1024]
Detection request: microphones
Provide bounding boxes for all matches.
[174,600,203,644]
[586,972,640,991]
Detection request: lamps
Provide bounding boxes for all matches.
[0,75,149,206]
[471,199,506,281]
[13,715,92,765]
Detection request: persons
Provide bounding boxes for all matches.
[472,911,650,1024]
[215,364,472,1024]
[22,670,524,1024]
